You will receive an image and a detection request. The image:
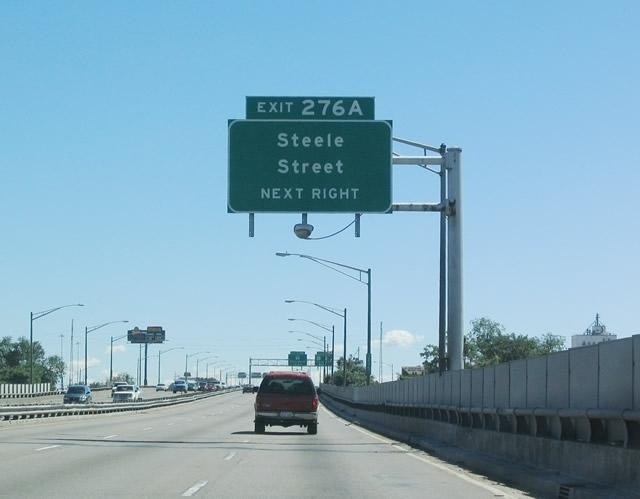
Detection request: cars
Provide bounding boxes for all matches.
[113,384,142,402]
[156,384,168,391]
[255,372,319,435]
[173,380,226,394]
[243,384,254,394]
[63,384,93,405]
[111,381,127,398]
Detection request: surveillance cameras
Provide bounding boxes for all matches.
[293,224,314,239]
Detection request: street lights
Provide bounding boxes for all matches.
[289,318,334,383]
[29,303,84,384]
[196,356,219,378]
[276,250,373,385]
[186,351,211,377]
[290,330,326,381]
[110,332,142,379]
[284,300,347,386]
[158,345,184,384]
[84,320,129,385]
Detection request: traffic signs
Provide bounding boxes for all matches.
[315,352,333,366]
[224,96,392,214]
[288,351,307,366]
